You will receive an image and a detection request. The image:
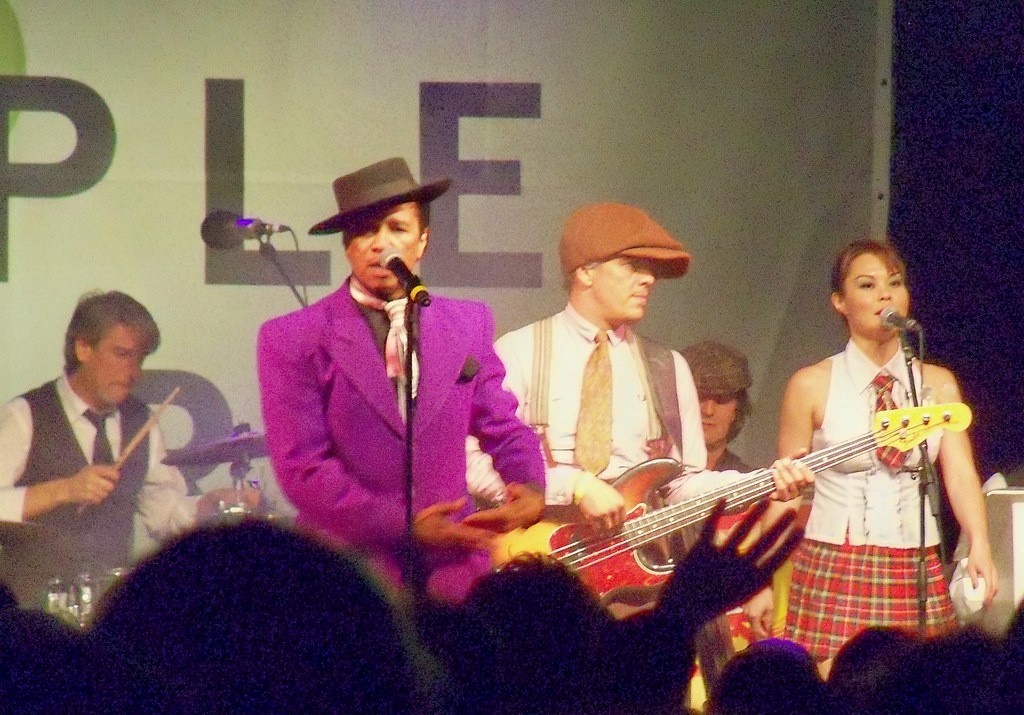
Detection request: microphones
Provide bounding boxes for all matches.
[200,210,289,249]
[881,307,922,334]
[380,247,431,307]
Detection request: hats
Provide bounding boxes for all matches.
[308,158,454,238]
[558,201,691,280]
[679,341,753,395]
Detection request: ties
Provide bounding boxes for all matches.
[349,272,419,423]
[575,329,613,477]
[873,376,913,467]
[83,407,114,465]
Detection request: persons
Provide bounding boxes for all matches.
[773,239,1003,681]
[680,339,773,715]
[0,291,262,606]
[0,496,1024,715]
[257,158,546,606]
[465,203,816,618]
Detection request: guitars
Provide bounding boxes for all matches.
[505,386,973,614]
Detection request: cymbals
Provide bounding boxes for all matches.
[0,520,79,605]
[159,431,274,467]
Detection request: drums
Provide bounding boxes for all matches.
[47,564,129,630]
